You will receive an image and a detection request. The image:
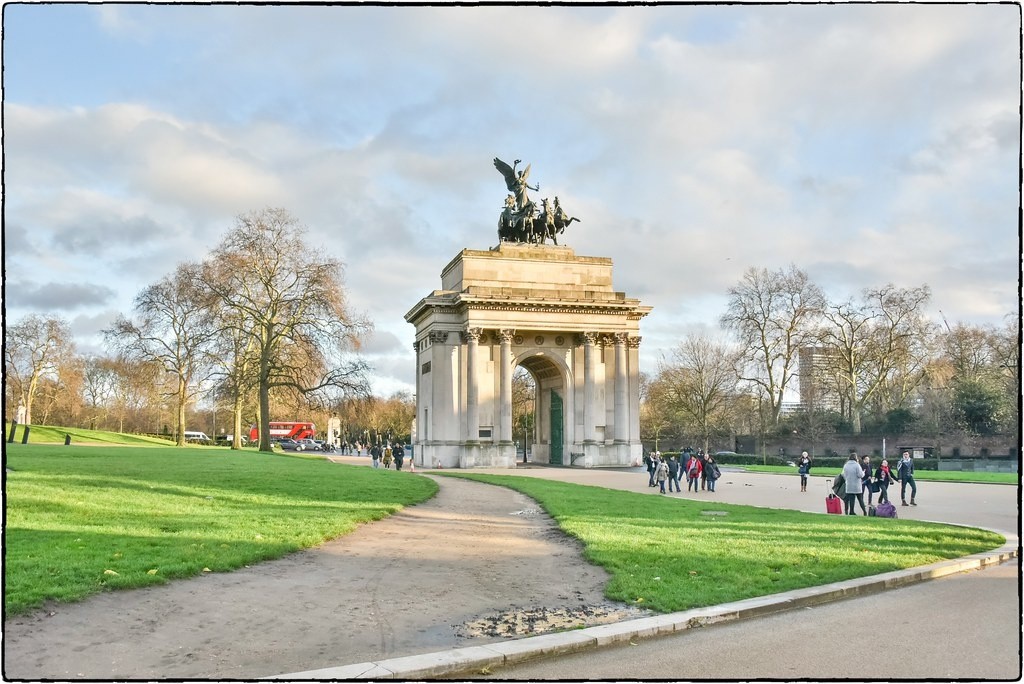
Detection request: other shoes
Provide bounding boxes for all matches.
[655,484,659,486]
[708,489,712,491]
[663,489,666,493]
[670,491,673,492]
[660,490,663,493]
[712,490,715,492]
[850,513,855,515]
[677,490,681,492]
[649,485,652,487]
[703,489,705,490]
[695,491,697,492]
[864,511,867,516]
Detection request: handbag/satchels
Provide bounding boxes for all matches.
[711,470,721,478]
[690,468,698,474]
[800,465,806,472]
[872,478,880,492]
[804,474,810,477]
[869,503,898,518]
[832,472,847,499]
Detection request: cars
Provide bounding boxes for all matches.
[180,432,328,453]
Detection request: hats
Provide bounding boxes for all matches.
[388,446,392,449]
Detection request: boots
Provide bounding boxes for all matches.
[902,498,909,506]
[804,486,806,491]
[801,485,803,492]
[910,497,917,505]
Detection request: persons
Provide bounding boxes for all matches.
[367,442,404,470]
[798,452,811,492]
[322,442,335,452]
[499,160,567,244]
[647,447,721,494]
[341,441,361,457]
[834,451,917,515]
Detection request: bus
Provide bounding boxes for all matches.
[248,422,315,443]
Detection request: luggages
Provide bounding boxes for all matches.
[825,480,842,514]
